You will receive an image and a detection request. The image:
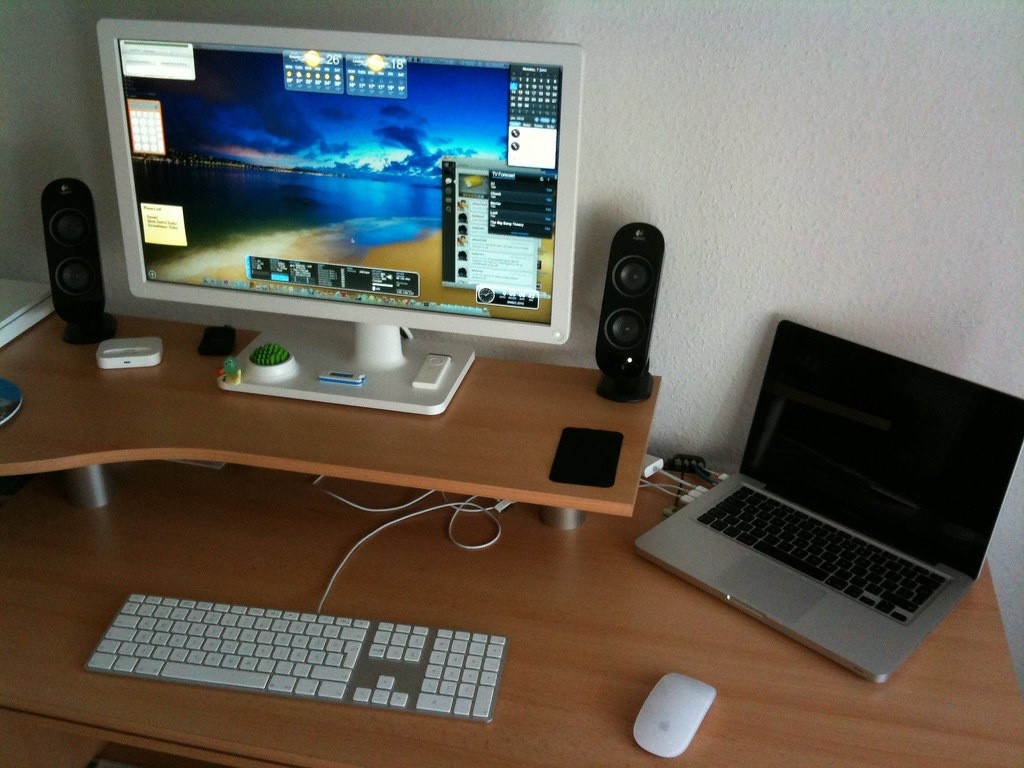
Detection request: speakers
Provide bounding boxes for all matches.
[595,222,665,403]
[42,177,117,346]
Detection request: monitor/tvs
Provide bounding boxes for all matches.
[96,19,585,416]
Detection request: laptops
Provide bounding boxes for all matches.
[634,320,1024,684]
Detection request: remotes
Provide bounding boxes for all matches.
[413,354,452,390]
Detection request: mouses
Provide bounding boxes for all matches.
[632,672,717,759]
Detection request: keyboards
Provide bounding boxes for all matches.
[83,590,510,723]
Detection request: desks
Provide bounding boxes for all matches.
[1,303,1024,768]
